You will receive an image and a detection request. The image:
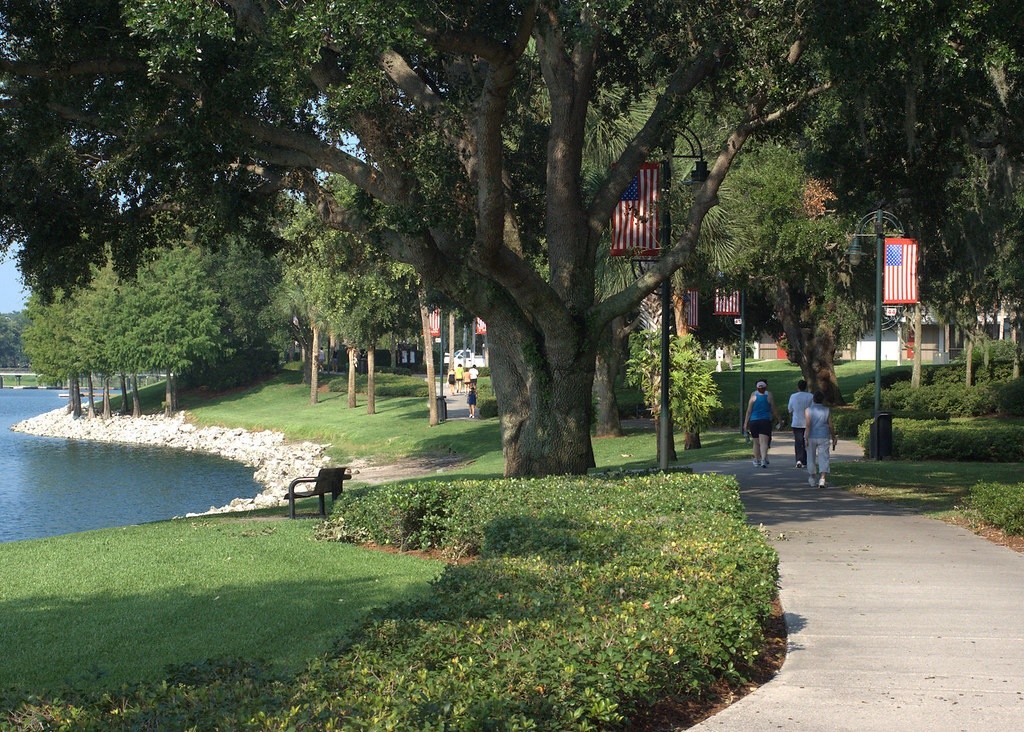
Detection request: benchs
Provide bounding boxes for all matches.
[284,467,351,520]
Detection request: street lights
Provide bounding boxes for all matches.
[656,154,711,471]
[843,234,883,461]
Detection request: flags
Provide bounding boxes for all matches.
[683,287,699,328]
[429,303,441,337]
[610,159,663,257]
[884,237,919,304]
[715,286,741,316]
[475,316,487,335]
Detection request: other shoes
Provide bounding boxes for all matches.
[765,459,769,465]
[801,464,807,468]
[752,461,760,468]
[818,479,826,488]
[472,416,475,419]
[469,414,472,418]
[795,461,803,468]
[760,460,767,468]
[808,476,816,487]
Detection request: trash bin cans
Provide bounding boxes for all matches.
[436,395,447,422]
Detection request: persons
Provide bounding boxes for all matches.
[447,364,479,395]
[788,380,813,468]
[804,391,836,488]
[467,387,476,419]
[744,379,784,467]
[319,347,339,372]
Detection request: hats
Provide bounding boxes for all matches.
[757,381,767,389]
